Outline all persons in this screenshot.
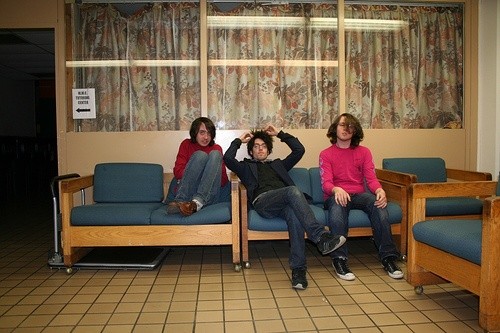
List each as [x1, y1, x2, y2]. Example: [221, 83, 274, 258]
[167, 117, 229, 217]
[222, 124, 346, 290]
[319, 113, 403, 281]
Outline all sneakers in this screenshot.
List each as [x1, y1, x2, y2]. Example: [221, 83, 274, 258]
[331, 256, 356, 281]
[316, 232, 346, 256]
[381, 257, 404, 279]
[291, 269, 308, 290]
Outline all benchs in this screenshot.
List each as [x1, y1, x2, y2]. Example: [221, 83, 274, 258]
[239, 168, 414, 270]
[58, 162, 242, 274]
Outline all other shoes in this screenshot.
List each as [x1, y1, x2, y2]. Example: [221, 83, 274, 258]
[167, 201, 181, 214]
[177, 200, 197, 216]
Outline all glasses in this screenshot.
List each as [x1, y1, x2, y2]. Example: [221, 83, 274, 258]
[253, 145, 268, 149]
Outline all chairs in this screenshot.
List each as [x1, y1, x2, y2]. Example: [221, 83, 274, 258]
[378, 158, 493, 248]
[407, 171, 500, 333]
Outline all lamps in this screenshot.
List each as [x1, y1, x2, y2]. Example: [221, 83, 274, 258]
[205, 15, 417, 32]
